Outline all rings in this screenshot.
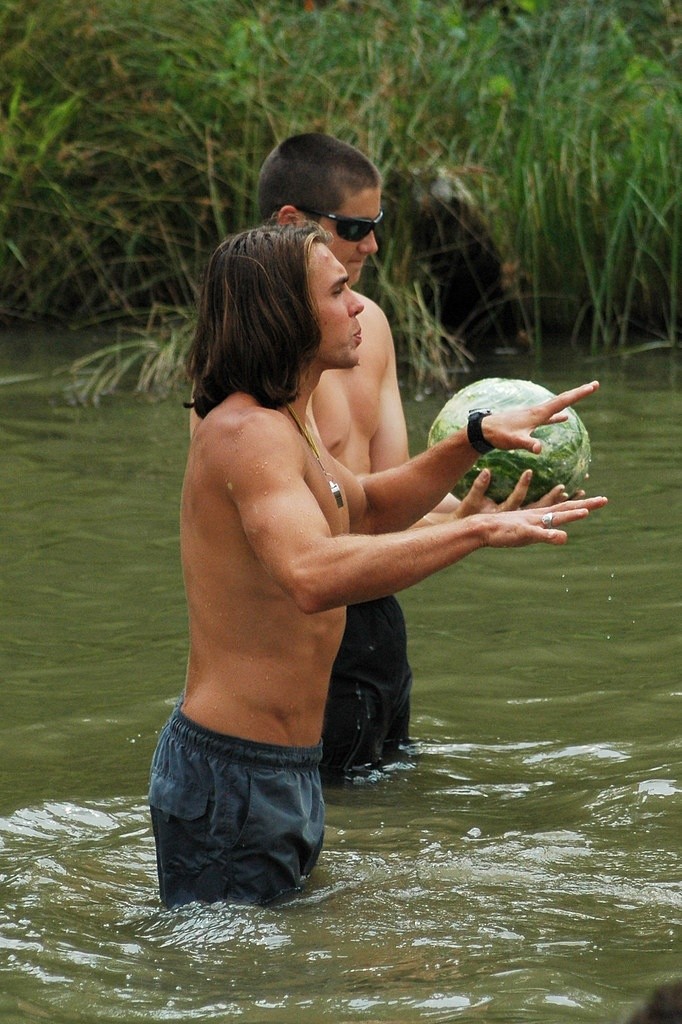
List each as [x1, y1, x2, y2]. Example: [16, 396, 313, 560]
[542, 512, 553, 529]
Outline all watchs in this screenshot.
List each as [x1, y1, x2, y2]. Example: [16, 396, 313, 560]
[466, 407, 496, 456]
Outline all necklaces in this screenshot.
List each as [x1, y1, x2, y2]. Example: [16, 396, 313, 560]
[286, 402, 344, 508]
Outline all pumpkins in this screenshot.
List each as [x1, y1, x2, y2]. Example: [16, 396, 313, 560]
[425, 379, 592, 508]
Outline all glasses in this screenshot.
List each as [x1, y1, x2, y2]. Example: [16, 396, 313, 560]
[276, 203, 383, 243]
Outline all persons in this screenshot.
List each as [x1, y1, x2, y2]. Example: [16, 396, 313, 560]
[190, 133, 587, 777]
[148, 223, 608, 910]
